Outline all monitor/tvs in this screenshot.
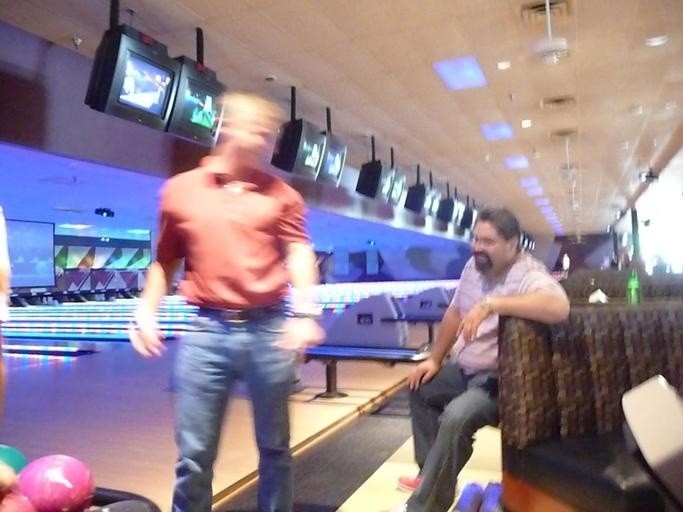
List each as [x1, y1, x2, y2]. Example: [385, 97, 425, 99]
[83, 21, 182, 131]
[270, 118, 328, 182]
[436, 199, 465, 226]
[460, 206, 478, 231]
[165, 54, 230, 149]
[519, 231, 536, 251]
[319, 130, 348, 188]
[355, 160, 406, 206]
[404, 184, 442, 217]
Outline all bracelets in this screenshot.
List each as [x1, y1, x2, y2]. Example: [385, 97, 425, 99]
[480, 296, 494, 312]
[288, 314, 318, 320]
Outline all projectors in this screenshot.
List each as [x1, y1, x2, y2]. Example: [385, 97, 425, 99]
[94, 209, 115, 218]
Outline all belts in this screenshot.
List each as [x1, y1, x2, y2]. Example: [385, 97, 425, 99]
[219, 308, 251, 324]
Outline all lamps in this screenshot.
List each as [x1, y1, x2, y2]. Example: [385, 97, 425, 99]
[521, 0, 584, 245]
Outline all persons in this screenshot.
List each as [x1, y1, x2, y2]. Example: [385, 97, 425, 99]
[397, 208, 570, 512]
[0, 206, 12, 423]
[127, 89, 318, 512]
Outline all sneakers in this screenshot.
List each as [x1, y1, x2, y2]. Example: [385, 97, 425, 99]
[398, 475, 423, 490]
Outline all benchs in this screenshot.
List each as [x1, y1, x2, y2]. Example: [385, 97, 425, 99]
[305, 345, 431, 398]
[380, 316, 442, 345]
